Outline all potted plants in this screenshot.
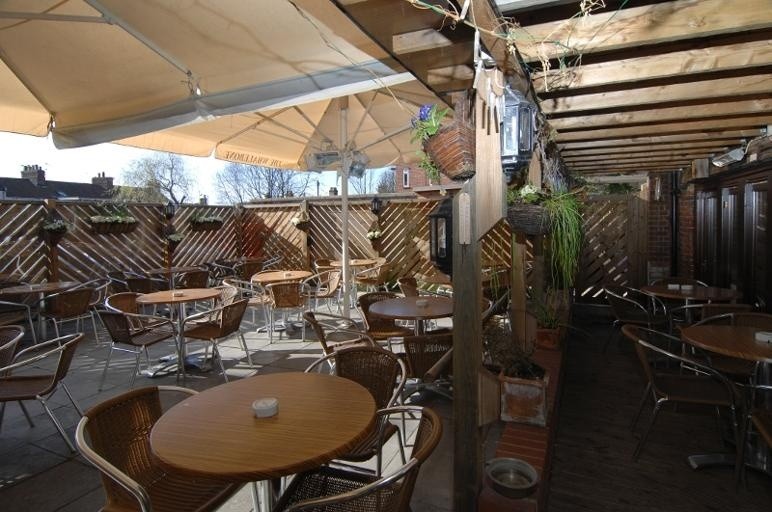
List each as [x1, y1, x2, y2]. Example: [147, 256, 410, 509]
[527, 283, 593, 350]
[484, 321, 552, 427]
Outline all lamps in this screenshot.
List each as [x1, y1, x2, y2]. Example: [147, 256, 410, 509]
[371, 194, 381, 214]
[502, 85, 534, 179]
[711, 147, 744, 169]
[428, 195, 454, 278]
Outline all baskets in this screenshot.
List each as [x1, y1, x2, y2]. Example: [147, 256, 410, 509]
[424, 97, 476, 181]
[507, 193, 562, 236]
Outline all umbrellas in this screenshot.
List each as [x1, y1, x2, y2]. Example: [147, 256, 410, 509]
[0, 1, 415, 151]
[108, 79, 450, 328]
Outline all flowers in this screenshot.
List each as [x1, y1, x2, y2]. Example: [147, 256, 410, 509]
[408, 102, 449, 195]
[43, 216, 223, 242]
[291, 217, 305, 227]
[509, 182, 587, 300]
[366, 230, 382, 240]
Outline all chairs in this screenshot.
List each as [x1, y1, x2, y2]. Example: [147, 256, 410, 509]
[176, 286, 242, 366]
[272, 405, 442, 512]
[0, 331, 81, 495]
[173, 298, 251, 386]
[95, 309, 177, 394]
[107, 289, 176, 374]
[1, 256, 354, 348]
[72, 382, 261, 512]
[354, 257, 508, 378]
[392, 332, 450, 423]
[280, 345, 407, 508]
[303, 311, 416, 420]
[604, 276, 772, 494]
